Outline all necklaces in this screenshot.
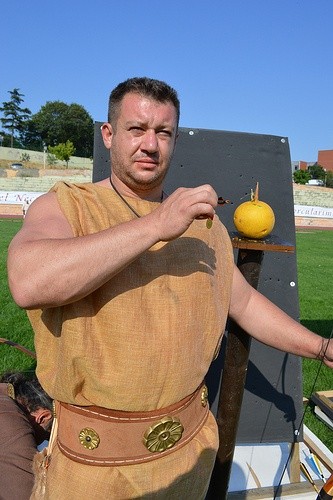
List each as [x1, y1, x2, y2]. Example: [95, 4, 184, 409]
[108, 177, 164, 219]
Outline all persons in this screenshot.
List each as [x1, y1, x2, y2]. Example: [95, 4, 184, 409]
[7, 77, 333, 500]
[0, 360, 53, 500]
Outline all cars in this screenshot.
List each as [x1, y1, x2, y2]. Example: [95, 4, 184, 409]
[10, 163, 24, 171]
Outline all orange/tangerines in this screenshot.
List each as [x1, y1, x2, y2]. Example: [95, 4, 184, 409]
[233, 180, 275, 238]
